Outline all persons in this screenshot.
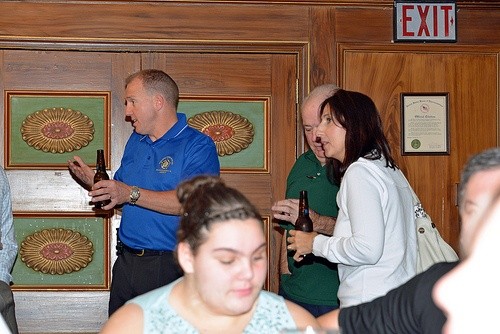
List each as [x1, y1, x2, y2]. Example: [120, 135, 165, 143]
[99, 175, 326, 334]
[271, 83, 341, 318]
[315, 147, 500, 334]
[1, 166, 20, 333]
[431, 193, 500, 333]
[287, 89, 459, 309]
[67, 69, 220, 319]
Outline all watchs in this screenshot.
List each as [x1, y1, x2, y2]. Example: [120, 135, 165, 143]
[130, 185, 141, 205]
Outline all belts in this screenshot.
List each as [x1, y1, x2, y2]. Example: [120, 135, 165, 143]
[123, 244, 160, 256]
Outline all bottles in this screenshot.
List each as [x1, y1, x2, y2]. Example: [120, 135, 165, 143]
[295, 190, 313, 257]
[93, 149, 112, 210]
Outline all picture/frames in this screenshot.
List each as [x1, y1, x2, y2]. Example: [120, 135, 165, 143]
[177, 93, 271, 174]
[4, 89, 112, 170]
[10, 211, 111, 291]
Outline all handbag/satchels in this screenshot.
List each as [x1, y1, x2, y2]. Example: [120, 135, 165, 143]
[396, 166, 460, 275]
